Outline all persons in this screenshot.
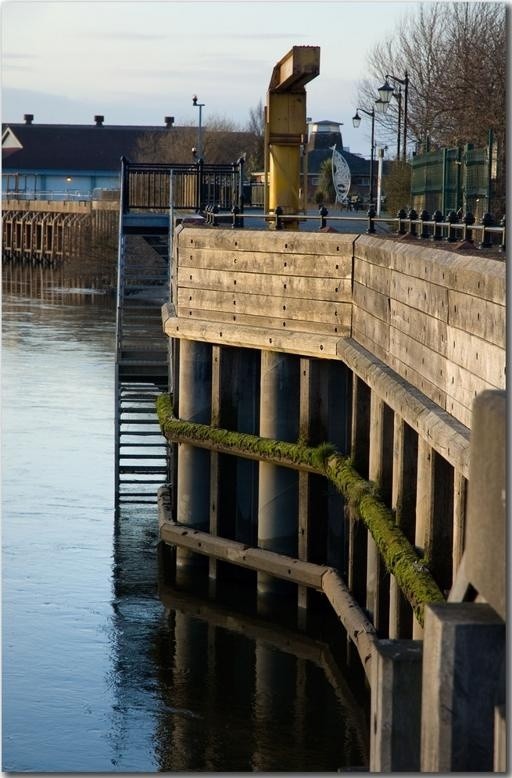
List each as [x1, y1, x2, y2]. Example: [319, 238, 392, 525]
[351, 198, 360, 213]
[346, 195, 353, 211]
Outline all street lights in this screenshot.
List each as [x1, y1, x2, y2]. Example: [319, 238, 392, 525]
[353, 107, 377, 202]
[190, 93, 205, 213]
[375, 71, 410, 170]
